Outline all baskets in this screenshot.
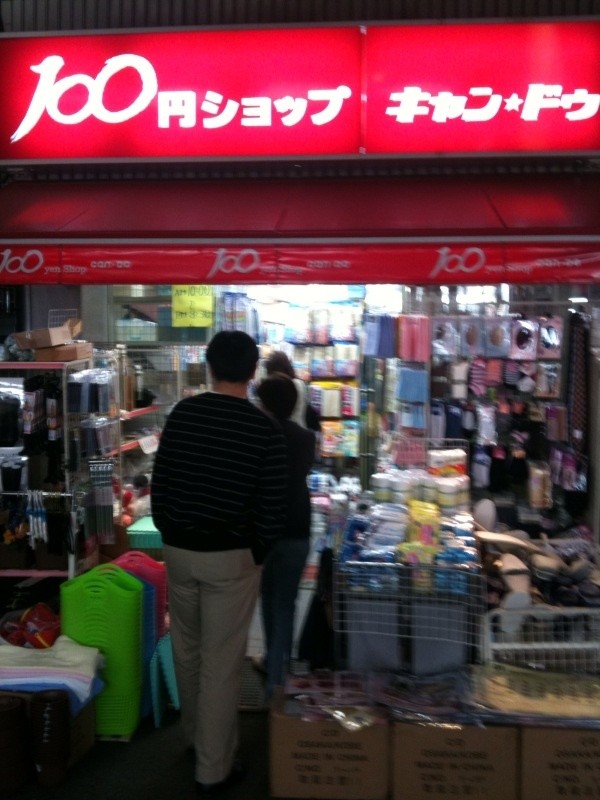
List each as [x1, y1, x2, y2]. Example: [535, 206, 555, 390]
[59, 550, 167, 736]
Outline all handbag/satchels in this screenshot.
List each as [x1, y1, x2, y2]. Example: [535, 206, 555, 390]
[303, 384, 320, 428]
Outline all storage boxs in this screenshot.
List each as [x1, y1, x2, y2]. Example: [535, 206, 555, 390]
[267, 684, 600, 800]
[15, 326, 92, 361]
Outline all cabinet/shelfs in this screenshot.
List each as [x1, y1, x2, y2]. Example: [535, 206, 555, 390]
[76, 342, 166, 559]
[0, 357, 98, 582]
[362, 314, 582, 542]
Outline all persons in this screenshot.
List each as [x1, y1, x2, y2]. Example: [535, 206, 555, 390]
[251, 351, 316, 683]
[148, 329, 289, 800]
